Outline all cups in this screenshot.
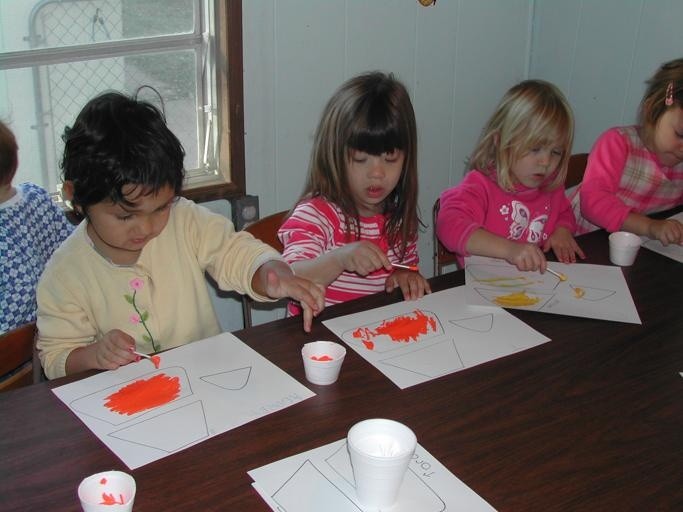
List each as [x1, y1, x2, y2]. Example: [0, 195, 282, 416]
[301, 341, 346, 386]
[78, 471, 136, 512]
[608, 232, 641, 266]
[347, 418, 417, 510]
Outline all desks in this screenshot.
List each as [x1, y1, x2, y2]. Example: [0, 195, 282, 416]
[1, 205, 682, 511]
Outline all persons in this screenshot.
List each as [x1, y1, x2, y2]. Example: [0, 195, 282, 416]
[0, 123, 76, 338]
[33, 85, 327, 382]
[564, 57, 683, 250]
[435, 76, 587, 276]
[275, 69, 433, 319]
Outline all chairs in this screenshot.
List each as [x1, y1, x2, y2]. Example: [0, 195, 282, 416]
[431, 151, 588, 278]
[239, 210, 295, 327]
